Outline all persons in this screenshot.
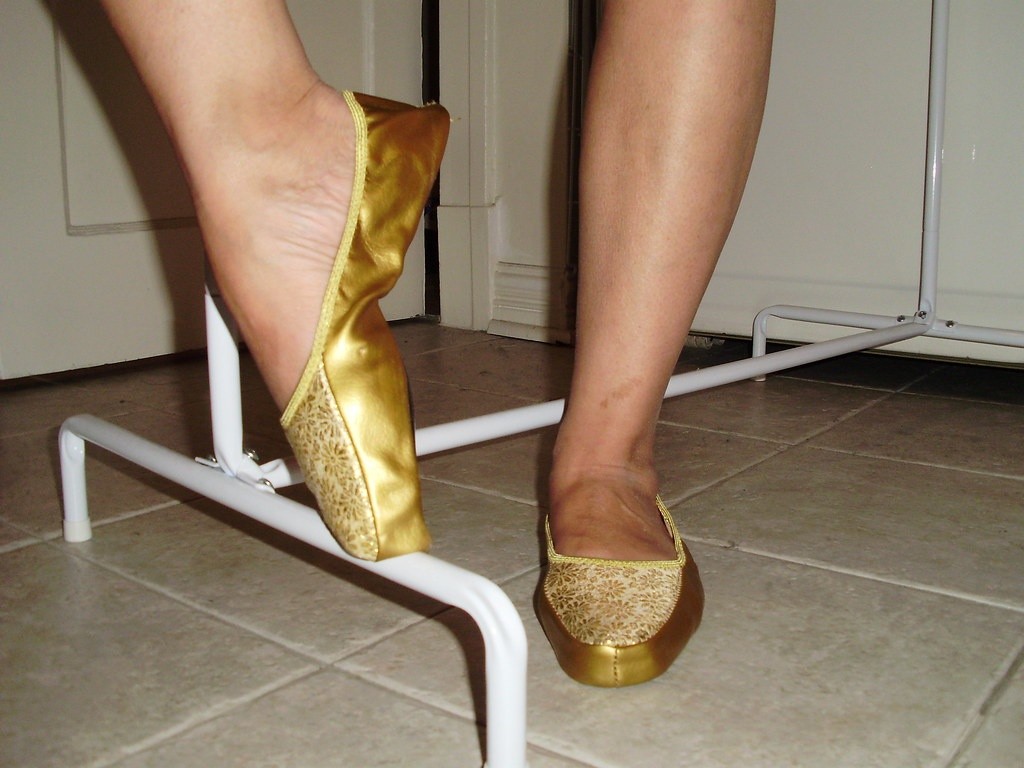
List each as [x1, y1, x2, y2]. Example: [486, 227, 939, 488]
[97, 1, 778, 688]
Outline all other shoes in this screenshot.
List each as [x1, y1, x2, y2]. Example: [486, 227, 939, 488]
[534, 485, 708, 693]
[281, 88, 452, 564]
